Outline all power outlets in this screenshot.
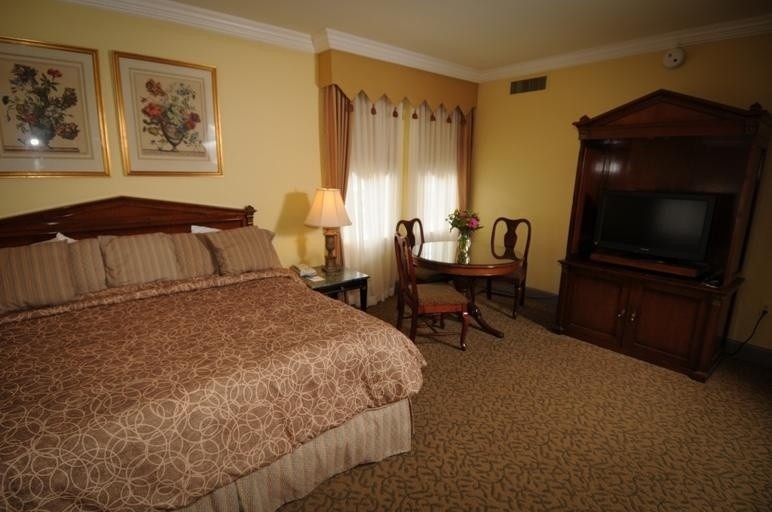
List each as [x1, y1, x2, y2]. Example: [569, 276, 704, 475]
[760, 301, 770, 317]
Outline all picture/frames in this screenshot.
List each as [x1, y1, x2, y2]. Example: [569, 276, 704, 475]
[1, 36, 111, 177]
[108, 50, 224, 176]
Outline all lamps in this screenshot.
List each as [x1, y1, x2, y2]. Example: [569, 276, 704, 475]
[304, 188, 353, 277]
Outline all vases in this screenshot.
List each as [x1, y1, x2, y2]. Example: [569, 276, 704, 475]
[458, 232, 472, 264]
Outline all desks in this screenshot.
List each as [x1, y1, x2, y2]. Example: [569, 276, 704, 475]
[410, 242, 524, 339]
[296, 268, 371, 312]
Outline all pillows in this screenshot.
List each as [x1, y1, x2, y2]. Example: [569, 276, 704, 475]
[171, 231, 219, 280]
[0, 239, 81, 316]
[207, 227, 277, 274]
[190, 224, 222, 236]
[62, 236, 114, 295]
[54, 232, 80, 245]
[98, 233, 175, 286]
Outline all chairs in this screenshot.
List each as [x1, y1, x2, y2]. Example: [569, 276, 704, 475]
[487, 217, 531, 320]
[392, 232, 470, 354]
[397, 218, 445, 330]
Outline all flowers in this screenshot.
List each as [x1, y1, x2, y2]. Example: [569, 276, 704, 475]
[450, 210, 481, 236]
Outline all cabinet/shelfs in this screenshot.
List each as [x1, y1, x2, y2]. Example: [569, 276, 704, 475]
[554, 89, 772, 384]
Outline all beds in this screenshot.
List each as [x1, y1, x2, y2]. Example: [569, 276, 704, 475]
[1, 198, 428, 511]
